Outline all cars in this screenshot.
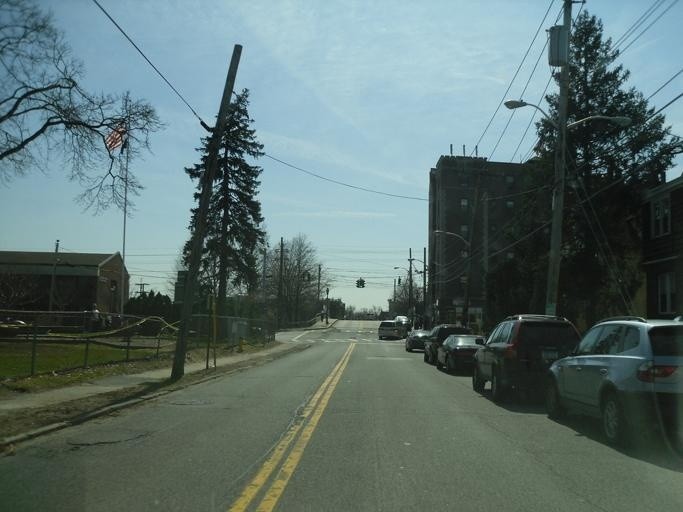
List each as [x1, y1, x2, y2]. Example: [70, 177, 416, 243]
[0, 316, 31, 338]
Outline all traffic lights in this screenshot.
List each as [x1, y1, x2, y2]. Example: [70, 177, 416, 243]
[355, 279, 360, 287]
[360, 279, 365, 288]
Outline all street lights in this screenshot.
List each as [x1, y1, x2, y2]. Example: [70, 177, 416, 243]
[503, 94, 631, 322]
[393, 254, 440, 330]
[434, 226, 497, 328]
